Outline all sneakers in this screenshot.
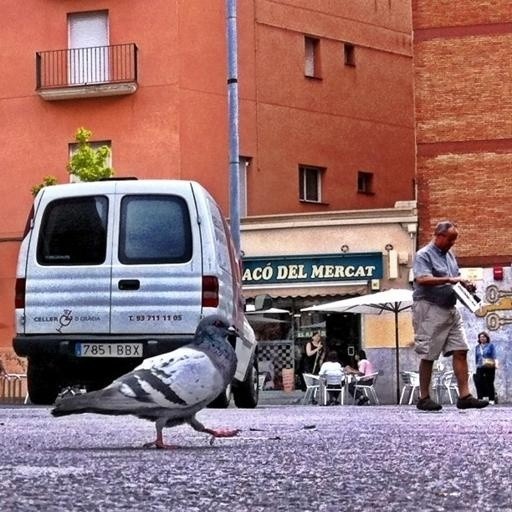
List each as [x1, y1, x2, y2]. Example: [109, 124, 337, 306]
[416, 395, 442, 411]
[357, 396, 369, 405]
[327, 399, 340, 406]
[456, 393, 490, 409]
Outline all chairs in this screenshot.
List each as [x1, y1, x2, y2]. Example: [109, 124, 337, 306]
[398, 368, 471, 405]
[301, 369, 380, 406]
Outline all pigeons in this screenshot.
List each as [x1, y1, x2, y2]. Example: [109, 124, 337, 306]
[50, 314, 244, 450]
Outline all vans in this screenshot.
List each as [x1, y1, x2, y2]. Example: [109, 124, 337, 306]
[9, 176, 262, 410]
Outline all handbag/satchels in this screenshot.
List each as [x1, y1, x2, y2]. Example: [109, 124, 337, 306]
[426, 286, 456, 307]
[482, 358, 495, 368]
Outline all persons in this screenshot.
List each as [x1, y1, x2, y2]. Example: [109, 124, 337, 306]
[411, 221, 488, 411]
[475, 330, 498, 405]
[298, 332, 373, 406]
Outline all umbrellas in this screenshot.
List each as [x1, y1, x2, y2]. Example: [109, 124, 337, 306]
[301, 288, 491, 405]
